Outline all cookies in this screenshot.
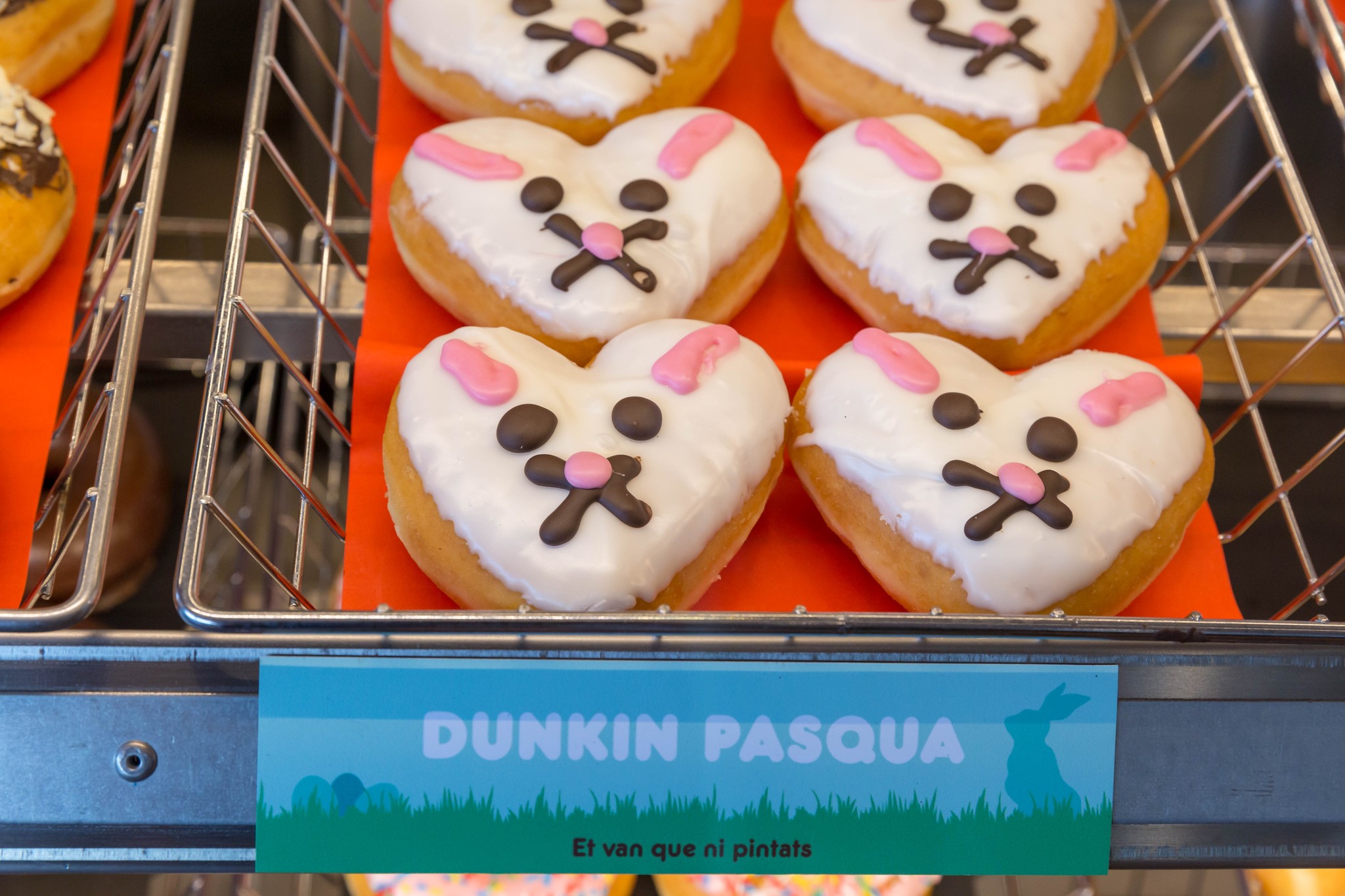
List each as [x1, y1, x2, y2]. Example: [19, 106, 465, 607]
[0, 0, 116, 312]
[381, 0, 1216, 621]
[343, 873, 947, 896]
